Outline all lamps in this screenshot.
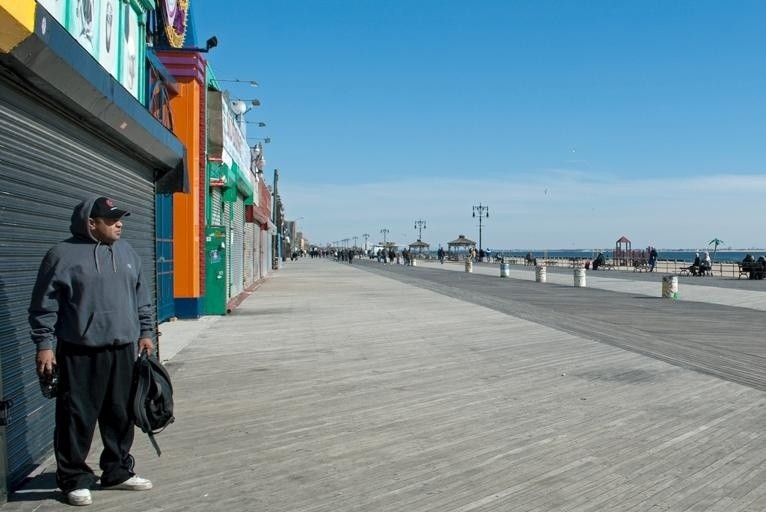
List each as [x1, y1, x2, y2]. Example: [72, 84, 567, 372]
[210, 79, 271, 144]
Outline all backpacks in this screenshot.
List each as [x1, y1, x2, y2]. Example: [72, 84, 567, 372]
[131, 347, 176, 459]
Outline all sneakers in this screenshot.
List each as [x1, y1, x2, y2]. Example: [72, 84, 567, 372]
[66, 487, 92, 506]
[100, 475, 153, 491]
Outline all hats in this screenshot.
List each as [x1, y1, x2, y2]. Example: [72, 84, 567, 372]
[89, 197, 131, 222]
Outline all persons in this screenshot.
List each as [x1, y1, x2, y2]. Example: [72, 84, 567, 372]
[698, 251, 712, 277]
[755, 256, 766, 280]
[287, 246, 538, 266]
[27, 194, 154, 505]
[689, 253, 701, 276]
[647, 246, 660, 273]
[743, 253, 756, 280]
[593, 251, 606, 270]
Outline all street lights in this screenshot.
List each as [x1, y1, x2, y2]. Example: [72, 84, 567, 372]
[472, 203, 488, 251]
[339, 227, 389, 248]
[414, 219, 426, 241]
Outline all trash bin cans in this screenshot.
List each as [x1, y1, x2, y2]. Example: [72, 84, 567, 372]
[273, 257, 282, 269]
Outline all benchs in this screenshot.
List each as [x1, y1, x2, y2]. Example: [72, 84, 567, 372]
[737, 262, 764, 280]
[680, 267, 714, 277]
